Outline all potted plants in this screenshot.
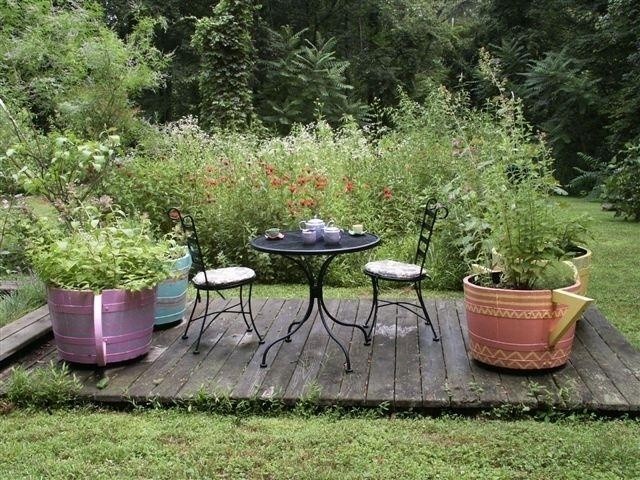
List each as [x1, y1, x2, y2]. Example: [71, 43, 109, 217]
[1, 182, 191, 370]
[434, 45, 595, 370]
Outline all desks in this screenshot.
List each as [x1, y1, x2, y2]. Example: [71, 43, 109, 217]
[250, 229, 380, 372]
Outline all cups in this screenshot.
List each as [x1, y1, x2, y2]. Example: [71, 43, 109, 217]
[301, 229, 317, 245]
[352, 225, 364, 233]
[265, 228, 281, 237]
[321, 226, 345, 245]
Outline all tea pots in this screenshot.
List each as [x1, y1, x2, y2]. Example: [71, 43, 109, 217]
[298, 214, 335, 242]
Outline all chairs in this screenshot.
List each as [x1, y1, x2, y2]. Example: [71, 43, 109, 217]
[167, 208, 265, 355]
[362, 198, 448, 341]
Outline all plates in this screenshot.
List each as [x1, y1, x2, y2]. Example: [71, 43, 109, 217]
[348, 230, 368, 236]
[264, 235, 287, 240]
